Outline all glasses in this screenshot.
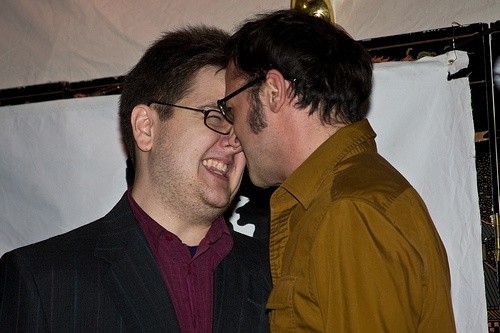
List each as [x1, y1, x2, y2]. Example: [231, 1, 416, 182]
[217, 77, 271, 121]
[145, 100, 232, 135]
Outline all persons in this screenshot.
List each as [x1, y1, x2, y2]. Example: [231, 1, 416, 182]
[224, 9, 457, 333]
[0, 24, 272, 333]
